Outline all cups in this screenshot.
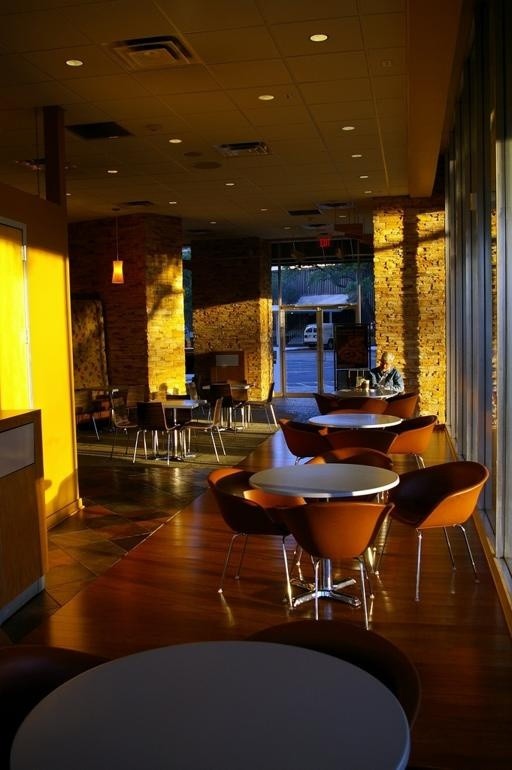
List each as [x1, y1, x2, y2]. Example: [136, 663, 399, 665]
[355, 377, 362, 388]
[362, 379, 370, 390]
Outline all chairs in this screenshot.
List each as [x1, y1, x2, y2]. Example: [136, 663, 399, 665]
[0, 643, 114, 770]
[245, 618, 424, 735]
[75, 379, 279, 469]
[312, 386, 421, 420]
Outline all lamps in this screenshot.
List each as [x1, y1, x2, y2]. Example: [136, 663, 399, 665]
[111, 215, 125, 285]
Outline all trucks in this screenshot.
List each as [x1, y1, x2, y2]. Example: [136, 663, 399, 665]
[303, 323, 333, 350]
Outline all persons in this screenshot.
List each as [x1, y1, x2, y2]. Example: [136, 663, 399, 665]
[361, 351, 404, 394]
